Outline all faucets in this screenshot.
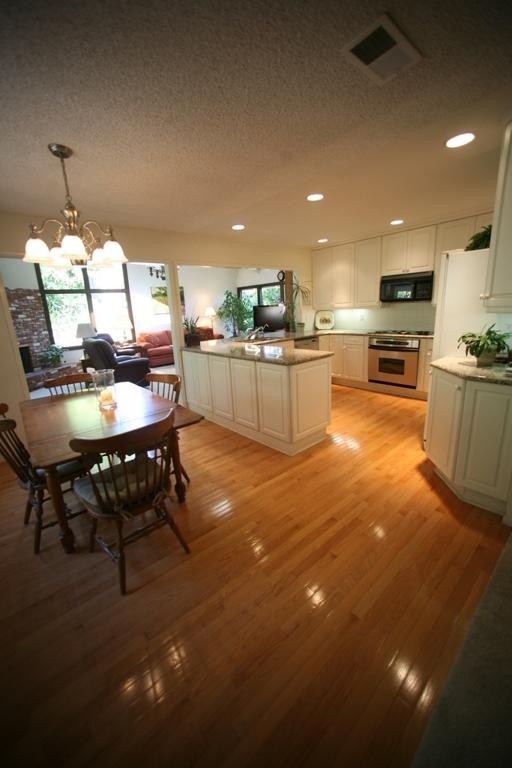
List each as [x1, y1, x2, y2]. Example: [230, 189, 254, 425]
[263, 324, 269, 329]
[247, 326, 263, 339]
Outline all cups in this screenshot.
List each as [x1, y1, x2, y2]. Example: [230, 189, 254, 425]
[91, 368, 117, 413]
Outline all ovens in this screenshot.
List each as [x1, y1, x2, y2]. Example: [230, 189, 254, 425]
[368, 336, 420, 391]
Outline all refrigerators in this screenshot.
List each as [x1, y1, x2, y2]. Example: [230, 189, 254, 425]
[433, 248, 511, 361]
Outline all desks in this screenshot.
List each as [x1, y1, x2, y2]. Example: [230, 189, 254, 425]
[18, 379, 206, 555]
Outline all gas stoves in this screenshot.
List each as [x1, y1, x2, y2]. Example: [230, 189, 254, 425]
[374, 330, 432, 335]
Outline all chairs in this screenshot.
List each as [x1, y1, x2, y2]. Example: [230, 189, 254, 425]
[82, 333, 151, 387]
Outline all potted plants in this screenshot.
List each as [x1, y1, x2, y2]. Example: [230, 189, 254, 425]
[456, 321, 511, 368]
[183, 315, 201, 346]
[279, 275, 315, 332]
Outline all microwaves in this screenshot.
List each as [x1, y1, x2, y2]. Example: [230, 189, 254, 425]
[379, 271, 434, 303]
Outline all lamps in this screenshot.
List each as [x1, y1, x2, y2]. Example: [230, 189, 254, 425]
[76, 323, 97, 355]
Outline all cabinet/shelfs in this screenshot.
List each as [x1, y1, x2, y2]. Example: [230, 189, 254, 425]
[430, 208, 494, 306]
[415, 338, 433, 401]
[355, 233, 381, 308]
[381, 221, 436, 277]
[309, 238, 355, 311]
[318, 333, 368, 391]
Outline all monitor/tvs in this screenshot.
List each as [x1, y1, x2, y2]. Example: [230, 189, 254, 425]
[253, 305, 286, 332]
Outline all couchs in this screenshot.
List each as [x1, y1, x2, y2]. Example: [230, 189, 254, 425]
[139, 325, 224, 369]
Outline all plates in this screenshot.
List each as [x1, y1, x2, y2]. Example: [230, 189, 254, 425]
[313, 310, 335, 330]
